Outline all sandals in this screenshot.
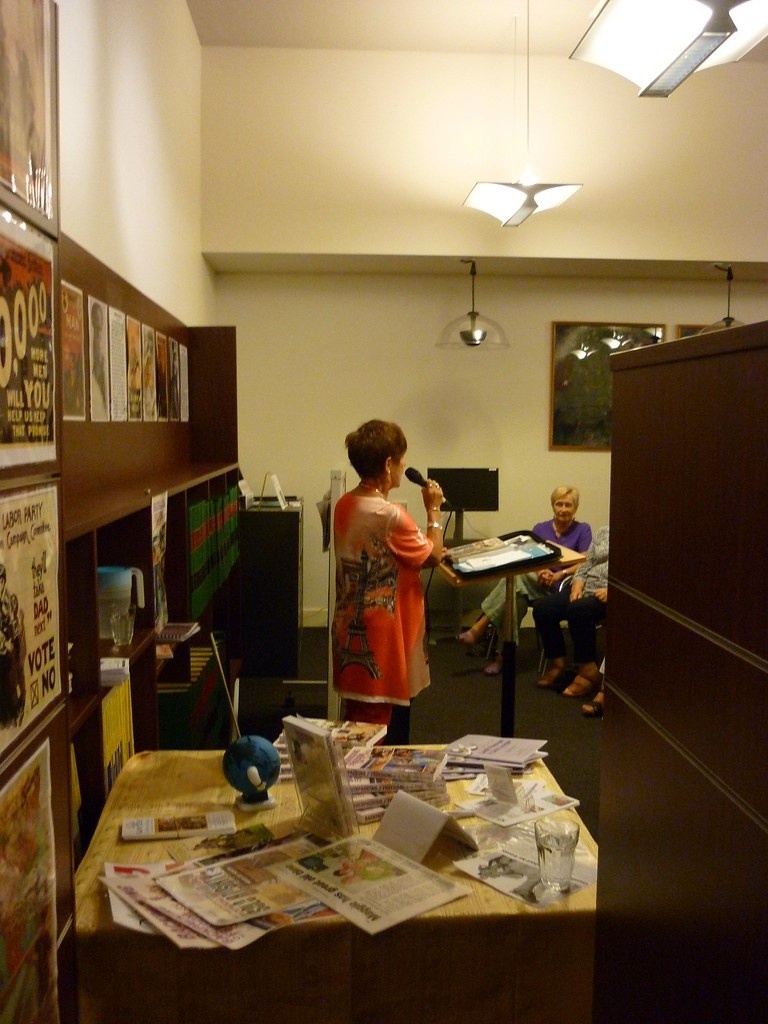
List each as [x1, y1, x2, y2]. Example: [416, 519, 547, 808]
[537, 664, 562, 689]
[582, 689, 605, 716]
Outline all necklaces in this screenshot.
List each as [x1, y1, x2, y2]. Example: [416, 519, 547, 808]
[359, 482, 384, 498]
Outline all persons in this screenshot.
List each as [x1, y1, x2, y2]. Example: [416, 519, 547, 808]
[532, 525, 610, 716]
[456, 486, 592, 677]
[331, 420, 443, 745]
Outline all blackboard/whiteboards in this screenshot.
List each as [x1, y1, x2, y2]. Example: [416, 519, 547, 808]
[328, 468, 342, 722]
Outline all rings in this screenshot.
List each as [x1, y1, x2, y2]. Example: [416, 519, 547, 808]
[426, 484, 432, 487]
[435, 483, 439, 488]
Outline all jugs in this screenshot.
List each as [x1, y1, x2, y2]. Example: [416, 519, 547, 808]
[96, 566, 145, 639]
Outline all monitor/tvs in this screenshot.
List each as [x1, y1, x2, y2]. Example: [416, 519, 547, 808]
[427, 468, 499, 511]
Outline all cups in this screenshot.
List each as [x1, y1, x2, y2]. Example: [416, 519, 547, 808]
[534, 817, 580, 892]
[108, 603, 136, 646]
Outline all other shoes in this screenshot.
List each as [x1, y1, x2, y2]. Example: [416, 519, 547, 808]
[458, 628, 480, 646]
[484, 660, 501, 674]
[562, 675, 600, 697]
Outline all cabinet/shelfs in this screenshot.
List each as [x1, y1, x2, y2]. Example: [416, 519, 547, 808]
[237, 495, 303, 677]
[58, 232, 234, 864]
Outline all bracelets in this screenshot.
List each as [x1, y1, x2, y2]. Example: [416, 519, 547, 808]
[562, 570, 567, 578]
[428, 507, 441, 514]
[427, 523, 443, 530]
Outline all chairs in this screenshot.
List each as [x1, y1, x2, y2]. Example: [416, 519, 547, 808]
[484, 574, 604, 677]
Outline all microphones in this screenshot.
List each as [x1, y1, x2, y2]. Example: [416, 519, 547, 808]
[404, 467, 452, 510]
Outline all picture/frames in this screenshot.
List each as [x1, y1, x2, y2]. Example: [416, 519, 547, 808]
[549, 322, 663, 451]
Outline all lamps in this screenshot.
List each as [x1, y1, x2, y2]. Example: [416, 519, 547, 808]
[463, 0, 583, 228]
[460, 259, 486, 346]
[568, 0, 768, 99]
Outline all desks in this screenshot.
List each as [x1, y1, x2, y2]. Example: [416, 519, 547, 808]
[71, 750, 597, 1024]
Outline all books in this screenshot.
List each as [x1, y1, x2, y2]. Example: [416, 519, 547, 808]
[273, 712, 550, 840]
